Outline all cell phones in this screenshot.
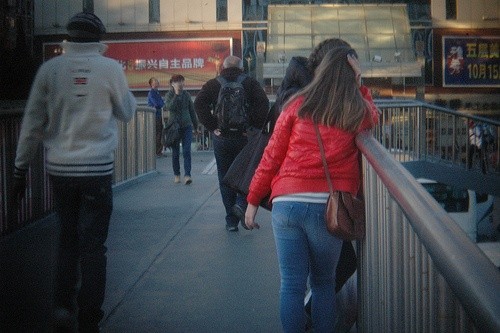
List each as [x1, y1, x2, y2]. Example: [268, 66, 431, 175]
[171, 82, 176, 87]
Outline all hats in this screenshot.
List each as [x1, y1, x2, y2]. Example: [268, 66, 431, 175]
[68, 12, 106, 38]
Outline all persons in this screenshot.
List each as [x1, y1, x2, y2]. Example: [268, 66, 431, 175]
[272, 39, 358, 333]
[462, 114, 489, 175]
[148, 77, 164, 154]
[11, 13, 137, 333]
[245, 46, 378, 333]
[165, 74, 197, 184]
[194, 56, 270, 231]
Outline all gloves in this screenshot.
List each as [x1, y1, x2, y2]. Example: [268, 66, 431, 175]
[9, 179, 26, 210]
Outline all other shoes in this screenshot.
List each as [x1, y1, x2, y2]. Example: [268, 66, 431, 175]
[174, 175, 180, 183]
[232, 202, 249, 230]
[226, 223, 238, 231]
[53, 306, 75, 329]
[184, 176, 192, 184]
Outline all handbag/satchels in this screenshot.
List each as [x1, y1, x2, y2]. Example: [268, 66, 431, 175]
[160, 122, 183, 147]
[324, 191, 365, 241]
[222, 103, 276, 212]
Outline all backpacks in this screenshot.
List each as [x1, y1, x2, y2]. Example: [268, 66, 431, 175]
[213, 75, 249, 137]
